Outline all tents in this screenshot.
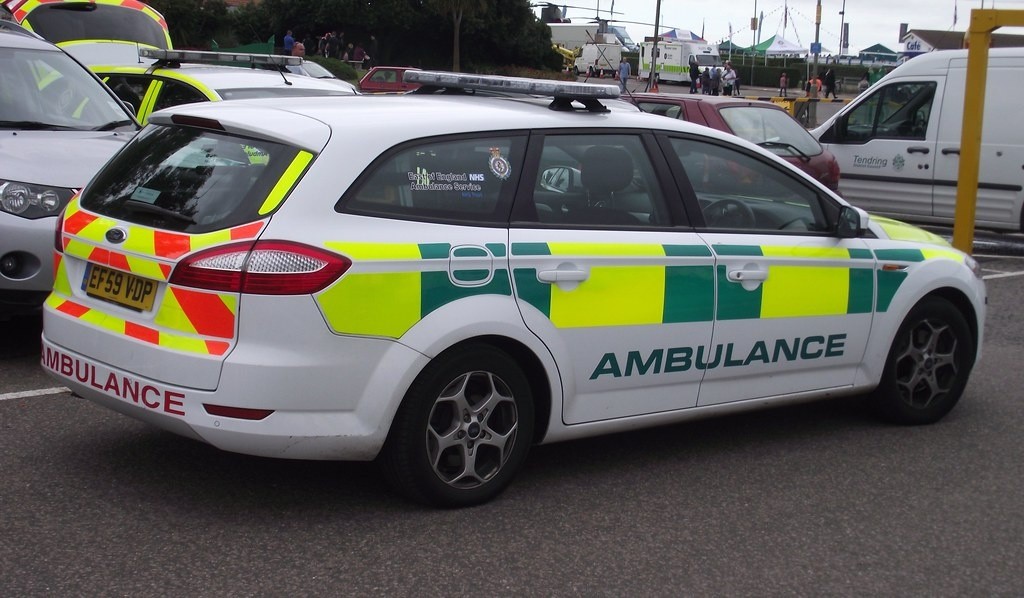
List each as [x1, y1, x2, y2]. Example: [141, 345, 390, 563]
[661, 28, 708, 43]
[718, 34, 809, 66]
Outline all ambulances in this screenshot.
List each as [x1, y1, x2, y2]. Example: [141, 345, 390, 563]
[34, 71, 990, 508]
[0, 0, 363, 174]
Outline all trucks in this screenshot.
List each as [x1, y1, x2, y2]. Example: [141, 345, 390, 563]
[638, 41, 727, 85]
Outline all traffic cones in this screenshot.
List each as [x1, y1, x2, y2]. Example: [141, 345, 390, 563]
[565, 64, 570, 73]
[599, 67, 605, 79]
[613, 68, 619, 81]
[585, 66, 590, 77]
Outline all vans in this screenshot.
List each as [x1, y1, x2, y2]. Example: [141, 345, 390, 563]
[573, 42, 622, 76]
[800, 44, 1024, 240]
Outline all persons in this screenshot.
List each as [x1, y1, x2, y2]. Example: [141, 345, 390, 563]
[805, 72, 821, 96]
[825, 68, 838, 98]
[689, 61, 740, 96]
[780, 73, 787, 97]
[858, 66, 887, 94]
[286, 42, 305, 75]
[618, 57, 631, 93]
[303, 31, 378, 69]
[284, 31, 294, 55]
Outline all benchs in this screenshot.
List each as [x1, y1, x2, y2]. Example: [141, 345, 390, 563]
[340, 60, 363, 71]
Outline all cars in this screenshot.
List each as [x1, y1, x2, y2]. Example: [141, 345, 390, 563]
[1, 19, 147, 317]
[359, 66, 427, 95]
[618, 91, 846, 208]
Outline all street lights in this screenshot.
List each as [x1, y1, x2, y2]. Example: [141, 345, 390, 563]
[838, 9, 846, 57]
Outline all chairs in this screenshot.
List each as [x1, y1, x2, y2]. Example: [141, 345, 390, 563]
[577, 146, 640, 227]
